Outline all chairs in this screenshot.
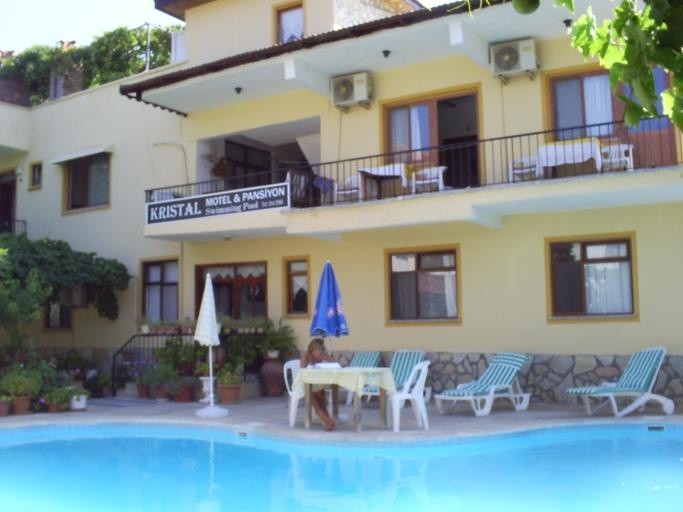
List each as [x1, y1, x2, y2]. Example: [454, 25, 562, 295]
[564, 348, 675, 419]
[434, 351, 531, 417]
[151, 169, 308, 217]
[386, 360, 431, 433]
[325, 350, 432, 409]
[284, 359, 332, 427]
[509, 137, 634, 183]
[335, 163, 448, 203]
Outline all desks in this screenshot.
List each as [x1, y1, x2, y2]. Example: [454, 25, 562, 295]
[294, 367, 397, 433]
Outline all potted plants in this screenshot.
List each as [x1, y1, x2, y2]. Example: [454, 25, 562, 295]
[0, 312, 292, 418]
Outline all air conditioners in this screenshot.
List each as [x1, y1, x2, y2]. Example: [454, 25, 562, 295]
[329, 67, 375, 111]
[487, 34, 540, 81]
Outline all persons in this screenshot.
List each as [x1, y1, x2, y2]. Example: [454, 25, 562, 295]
[301, 336, 351, 433]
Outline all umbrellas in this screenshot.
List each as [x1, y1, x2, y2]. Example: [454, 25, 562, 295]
[194, 273, 227, 417]
[310, 261, 350, 424]
